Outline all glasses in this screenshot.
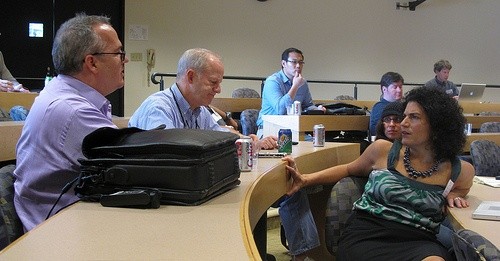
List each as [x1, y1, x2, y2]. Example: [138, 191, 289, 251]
[83, 50, 126, 64]
[384, 118, 404, 122]
[286, 60, 305, 64]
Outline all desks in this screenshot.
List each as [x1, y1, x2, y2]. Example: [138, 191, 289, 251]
[0, 116, 131, 161]
[445, 176, 500, 252]
[0, 91, 40, 117]
[462, 132, 500, 152]
[0, 140, 360, 261]
[260, 114, 500, 141]
[209, 97, 500, 119]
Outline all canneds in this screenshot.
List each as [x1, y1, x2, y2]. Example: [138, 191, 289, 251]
[234, 137, 253, 172]
[277, 128, 292, 155]
[312, 124, 325, 147]
[293, 100, 302, 115]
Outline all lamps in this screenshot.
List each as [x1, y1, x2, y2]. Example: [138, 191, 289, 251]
[396, 0, 428, 11]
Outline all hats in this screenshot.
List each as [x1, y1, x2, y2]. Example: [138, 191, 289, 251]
[382, 98, 407, 117]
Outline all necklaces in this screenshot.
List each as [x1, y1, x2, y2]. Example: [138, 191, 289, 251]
[403, 146, 440, 178]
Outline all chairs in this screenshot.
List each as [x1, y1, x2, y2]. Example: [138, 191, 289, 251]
[469, 139, 500, 177]
[325, 175, 369, 260]
[9, 105, 30, 121]
[449, 229, 500, 261]
[479, 122, 500, 132]
[0, 165, 24, 249]
[232, 88, 260, 98]
[478, 111, 500, 117]
[333, 95, 356, 100]
[241, 108, 260, 137]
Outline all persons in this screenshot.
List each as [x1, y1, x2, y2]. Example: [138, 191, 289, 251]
[0, 50, 31, 92]
[376, 101, 407, 142]
[368, 71, 404, 142]
[129, 47, 277, 261]
[205, 103, 278, 149]
[255, 47, 326, 141]
[280, 86, 475, 260]
[423, 59, 460, 100]
[13, 14, 129, 235]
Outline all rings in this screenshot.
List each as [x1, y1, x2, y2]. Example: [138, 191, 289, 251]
[292, 162, 297, 168]
[455, 196, 461, 201]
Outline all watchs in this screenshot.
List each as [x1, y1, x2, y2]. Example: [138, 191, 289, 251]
[223, 111, 232, 122]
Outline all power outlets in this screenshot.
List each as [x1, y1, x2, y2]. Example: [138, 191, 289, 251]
[131, 53, 142, 61]
